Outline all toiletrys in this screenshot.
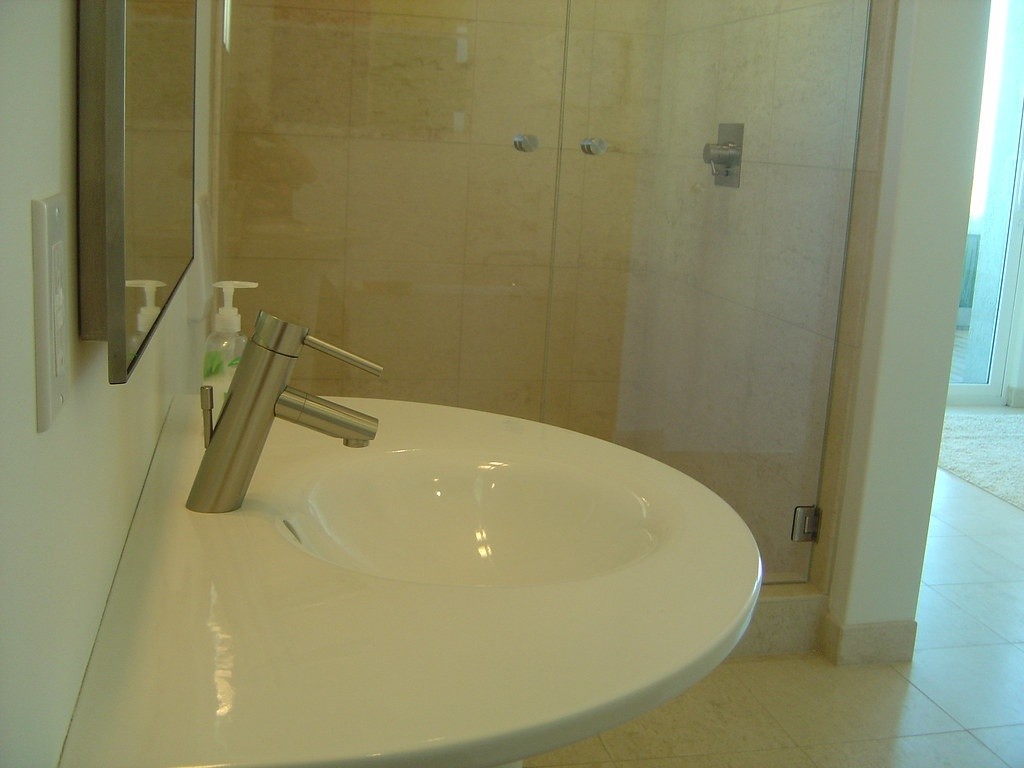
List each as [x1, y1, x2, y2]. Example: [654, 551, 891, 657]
[124, 279, 170, 368]
[199, 279, 260, 429]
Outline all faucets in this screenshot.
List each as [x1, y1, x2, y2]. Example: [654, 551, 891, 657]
[184, 308, 386, 514]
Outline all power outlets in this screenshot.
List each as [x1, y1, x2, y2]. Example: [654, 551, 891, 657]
[33, 191, 70, 433]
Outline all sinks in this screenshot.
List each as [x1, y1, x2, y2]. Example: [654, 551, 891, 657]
[282, 445, 661, 592]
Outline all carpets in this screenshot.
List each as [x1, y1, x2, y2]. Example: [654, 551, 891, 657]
[937, 412, 1023, 514]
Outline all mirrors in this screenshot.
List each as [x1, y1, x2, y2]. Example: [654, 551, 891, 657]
[79, 0, 197, 388]
